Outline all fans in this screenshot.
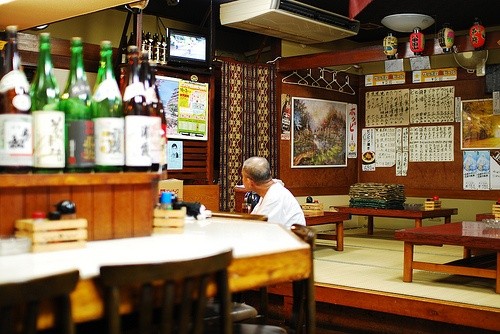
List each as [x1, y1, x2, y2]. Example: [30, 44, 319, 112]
[453, 50, 489, 76]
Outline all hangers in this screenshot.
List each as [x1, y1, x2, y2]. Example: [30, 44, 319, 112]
[282, 68, 356, 95]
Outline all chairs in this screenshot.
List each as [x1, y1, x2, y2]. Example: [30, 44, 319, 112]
[0, 251, 255, 334]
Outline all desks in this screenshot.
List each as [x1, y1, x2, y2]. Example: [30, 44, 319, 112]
[0, 168, 500, 334]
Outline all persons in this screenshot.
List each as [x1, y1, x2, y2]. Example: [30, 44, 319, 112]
[241, 157, 306, 229]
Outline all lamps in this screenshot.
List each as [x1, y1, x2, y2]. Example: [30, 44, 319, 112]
[381, 0, 435, 32]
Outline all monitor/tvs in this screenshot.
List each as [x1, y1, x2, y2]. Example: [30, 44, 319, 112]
[167, 28, 208, 67]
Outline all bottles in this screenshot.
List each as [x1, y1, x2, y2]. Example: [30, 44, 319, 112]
[138, 50, 169, 172]
[0, 26, 36, 175]
[60, 36, 97, 174]
[29, 33, 71, 175]
[128, 30, 174, 66]
[90, 39, 126, 172]
[119, 45, 157, 172]
[159, 192, 173, 211]
[31, 199, 78, 251]
[306, 196, 318, 203]
